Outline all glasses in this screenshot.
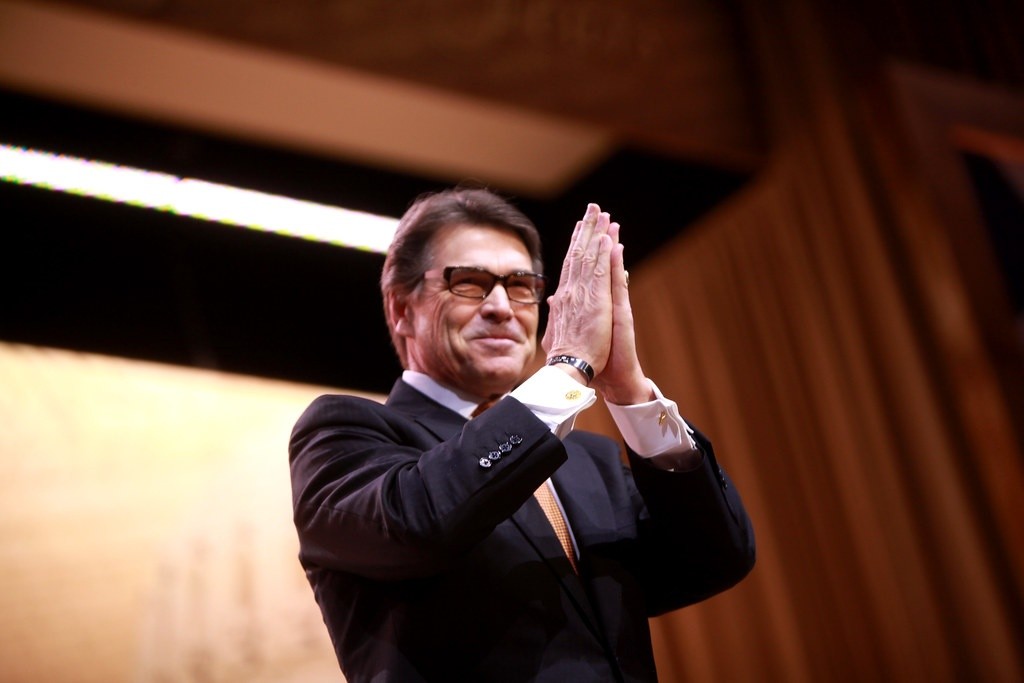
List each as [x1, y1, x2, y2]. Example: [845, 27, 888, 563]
[406, 266, 544, 303]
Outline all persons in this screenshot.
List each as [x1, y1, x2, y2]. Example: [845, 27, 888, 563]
[289, 191, 756, 683]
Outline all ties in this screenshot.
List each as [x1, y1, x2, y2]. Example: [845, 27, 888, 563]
[472, 399, 579, 576]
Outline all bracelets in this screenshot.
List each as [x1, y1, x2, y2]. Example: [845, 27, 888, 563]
[545, 355, 595, 386]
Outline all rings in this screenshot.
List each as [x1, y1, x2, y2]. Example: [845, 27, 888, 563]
[625, 270, 629, 284]
